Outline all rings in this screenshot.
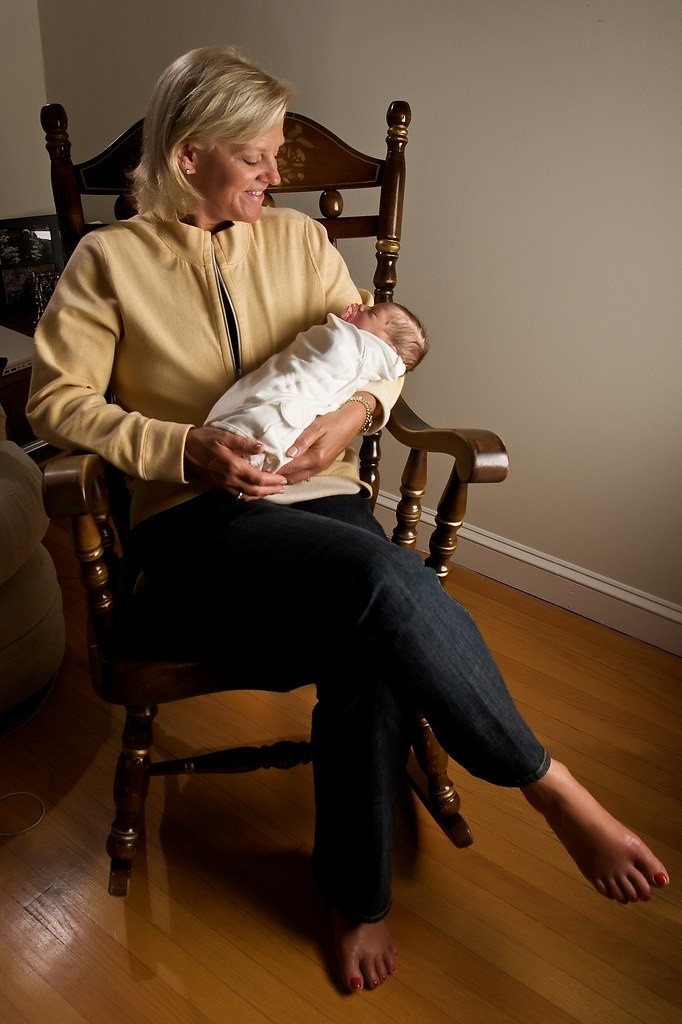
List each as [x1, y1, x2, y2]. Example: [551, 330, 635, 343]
[235, 491, 243, 500]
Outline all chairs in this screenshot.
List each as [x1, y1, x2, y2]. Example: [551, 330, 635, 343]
[41, 101, 511, 861]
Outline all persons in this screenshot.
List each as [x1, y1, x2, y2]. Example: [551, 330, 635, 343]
[200, 300, 430, 476]
[23, 43, 672, 994]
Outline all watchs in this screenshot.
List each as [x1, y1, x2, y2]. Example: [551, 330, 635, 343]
[346, 396, 373, 436]
